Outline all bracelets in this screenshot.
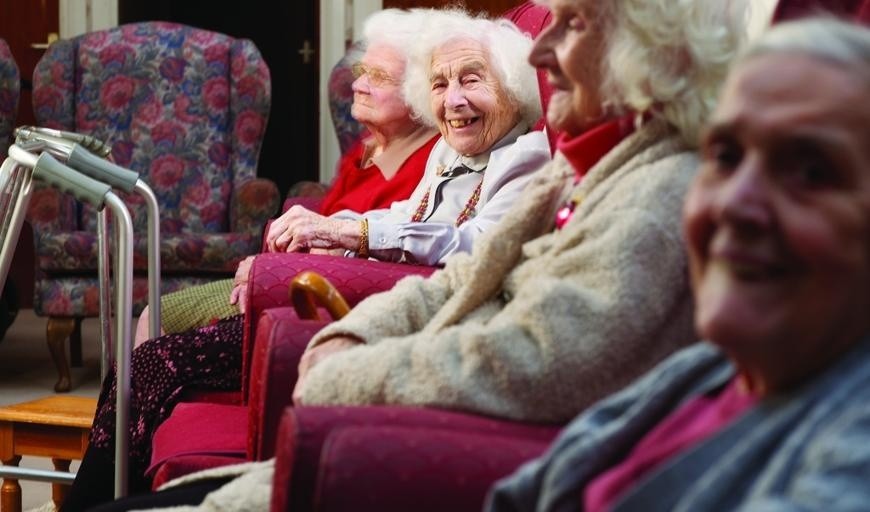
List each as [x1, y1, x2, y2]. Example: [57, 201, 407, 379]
[360, 215, 370, 258]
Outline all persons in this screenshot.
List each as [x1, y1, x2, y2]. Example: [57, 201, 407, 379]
[100, 1, 745, 511]
[61, 19, 551, 512]
[485, 15, 870, 512]
[135, 8, 442, 348]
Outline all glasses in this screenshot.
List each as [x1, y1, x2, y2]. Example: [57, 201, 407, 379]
[352, 62, 406, 86]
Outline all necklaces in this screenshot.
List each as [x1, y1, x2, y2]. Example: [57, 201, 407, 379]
[556, 198, 575, 231]
[408, 185, 485, 228]
